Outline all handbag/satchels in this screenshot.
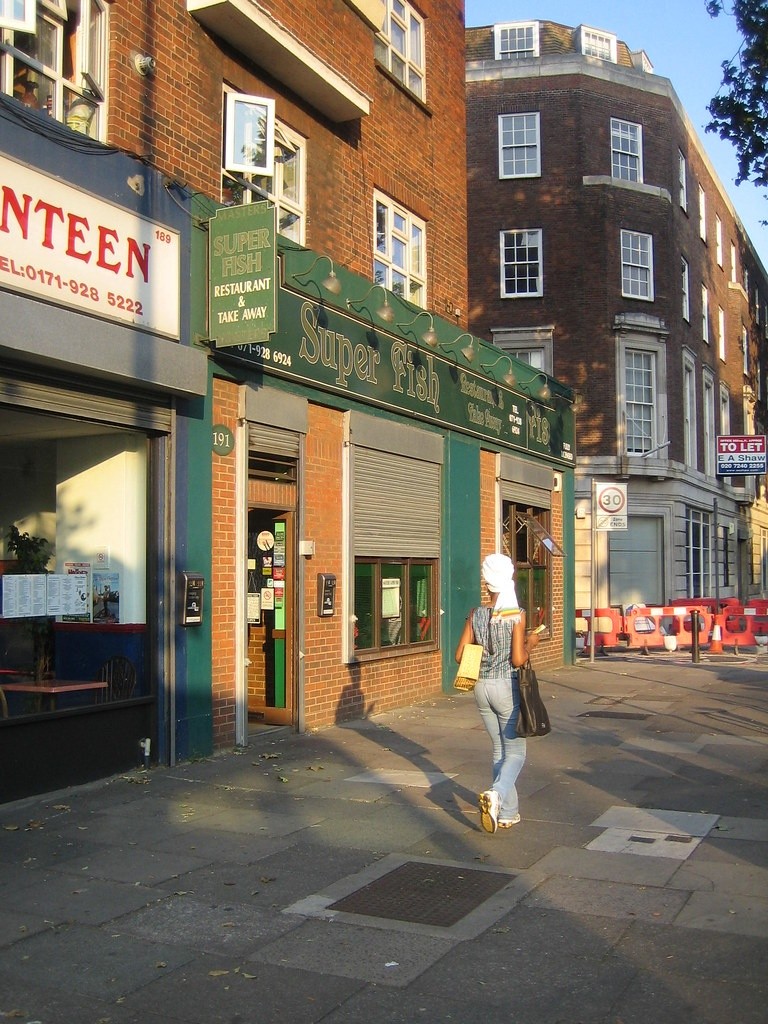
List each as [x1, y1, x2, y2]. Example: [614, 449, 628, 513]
[454, 644, 483, 691]
[514, 651, 552, 737]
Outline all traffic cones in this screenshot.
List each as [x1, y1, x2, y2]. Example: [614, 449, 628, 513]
[707, 618, 726, 655]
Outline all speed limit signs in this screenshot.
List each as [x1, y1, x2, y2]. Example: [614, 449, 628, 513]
[595, 482, 628, 532]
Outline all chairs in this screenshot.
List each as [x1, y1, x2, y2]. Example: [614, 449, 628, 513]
[92, 656, 136, 703]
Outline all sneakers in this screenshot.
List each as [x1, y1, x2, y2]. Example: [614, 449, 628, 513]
[498, 813, 521, 828]
[479, 791, 499, 834]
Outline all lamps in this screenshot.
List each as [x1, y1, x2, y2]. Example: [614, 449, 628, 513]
[518, 373, 551, 400]
[554, 393, 582, 413]
[346, 285, 395, 324]
[481, 358, 515, 386]
[439, 333, 475, 363]
[396, 312, 438, 347]
[292, 255, 341, 295]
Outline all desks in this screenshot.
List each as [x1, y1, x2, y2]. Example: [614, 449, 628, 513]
[1, 680, 108, 713]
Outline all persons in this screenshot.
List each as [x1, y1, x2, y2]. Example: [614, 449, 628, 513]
[454, 553, 540, 833]
[103, 585, 109, 617]
